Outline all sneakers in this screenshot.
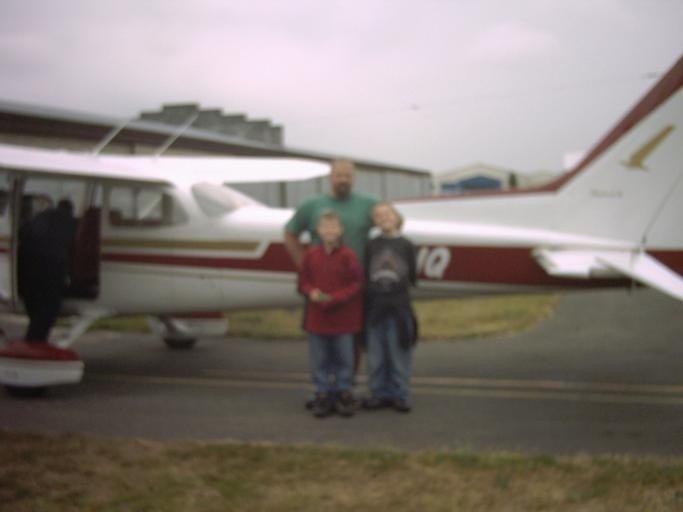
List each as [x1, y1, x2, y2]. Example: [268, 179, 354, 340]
[304, 390, 356, 417]
[392, 398, 410, 411]
[362, 395, 392, 409]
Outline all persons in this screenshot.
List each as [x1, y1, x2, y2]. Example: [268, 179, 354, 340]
[356, 197, 420, 414]
[17, 199, 77, 346]
[281, 157, 385, 412]
[292, 209, 366, 420]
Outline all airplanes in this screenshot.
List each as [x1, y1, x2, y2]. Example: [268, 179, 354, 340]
[0, 52, 683, 398]
